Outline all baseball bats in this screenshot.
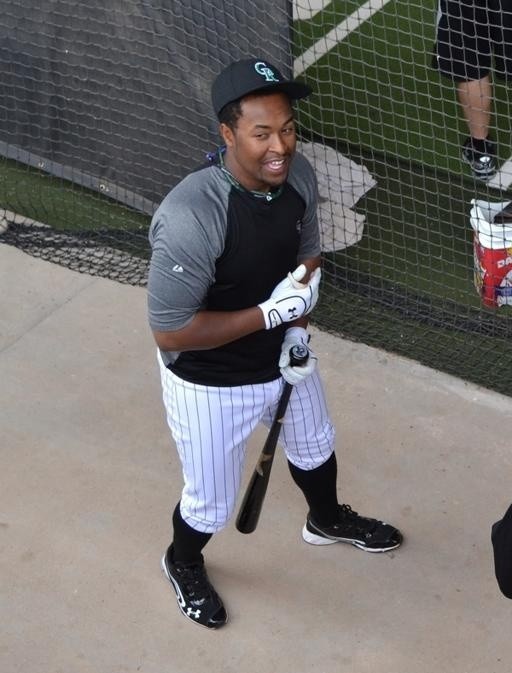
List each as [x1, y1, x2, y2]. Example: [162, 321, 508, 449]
[236, 345, 309, 534]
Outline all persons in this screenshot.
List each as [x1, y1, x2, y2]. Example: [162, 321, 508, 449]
[430, 0, 512, 175]
[146, 56, 403, 628]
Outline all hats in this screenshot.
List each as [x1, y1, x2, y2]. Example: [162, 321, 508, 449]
[211, 58, 313, 113]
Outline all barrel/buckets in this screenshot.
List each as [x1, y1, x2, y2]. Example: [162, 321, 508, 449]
[469, 198, 512, 308]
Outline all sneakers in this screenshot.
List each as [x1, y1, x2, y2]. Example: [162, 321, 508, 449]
[161, 542, 228, 628]
[461, 137, 496, 173]
[302, 504, 404, 552]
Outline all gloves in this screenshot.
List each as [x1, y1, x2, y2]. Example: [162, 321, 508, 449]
[279, 327, 318, 385]
[258, 263, 322, 330]
